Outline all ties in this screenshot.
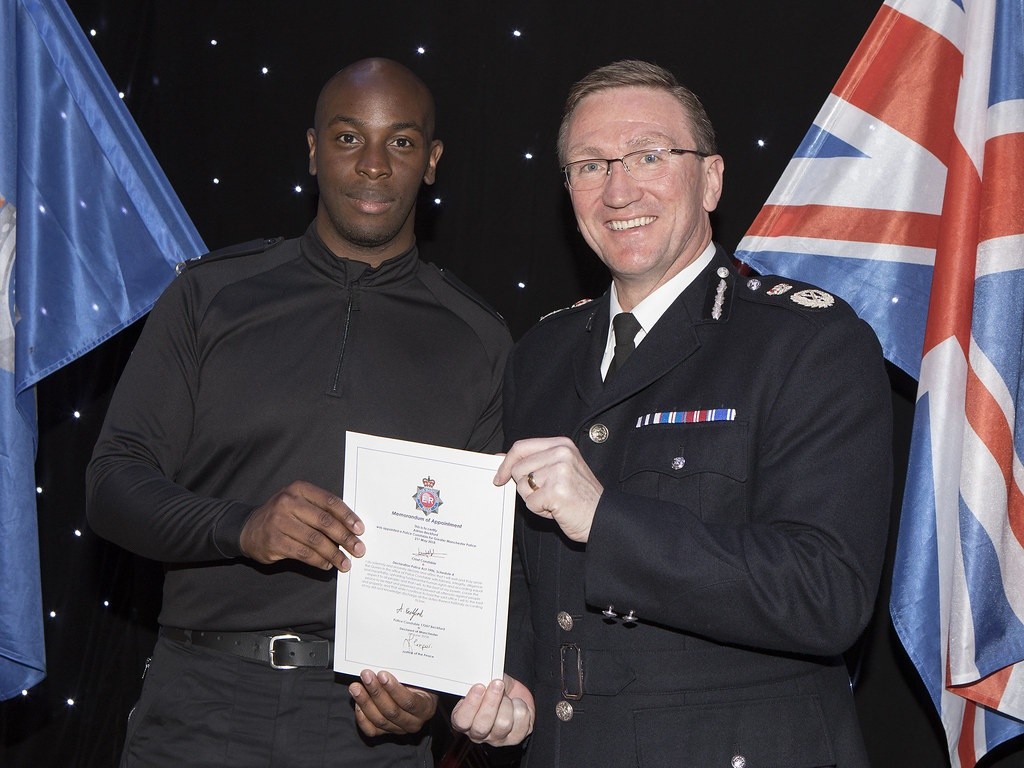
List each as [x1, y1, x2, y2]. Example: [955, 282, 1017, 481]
[603, 312, 642, 387]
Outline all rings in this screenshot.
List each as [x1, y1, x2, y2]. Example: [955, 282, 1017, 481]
[528, 472, 538, 491]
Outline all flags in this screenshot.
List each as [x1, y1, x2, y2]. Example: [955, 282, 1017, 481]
[734, 0, 1024, 768]
[0, 0, 210, 702]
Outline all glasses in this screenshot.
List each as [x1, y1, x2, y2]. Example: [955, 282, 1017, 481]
[562, 148, 712, 191]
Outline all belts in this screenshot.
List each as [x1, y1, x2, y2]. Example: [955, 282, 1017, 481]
[532, 643, 838, 701]
[161, 626, 335, 670]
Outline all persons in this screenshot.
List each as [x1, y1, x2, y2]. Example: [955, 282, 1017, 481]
[450, 61, 894, 768]
[84, 59, 516, 767]
